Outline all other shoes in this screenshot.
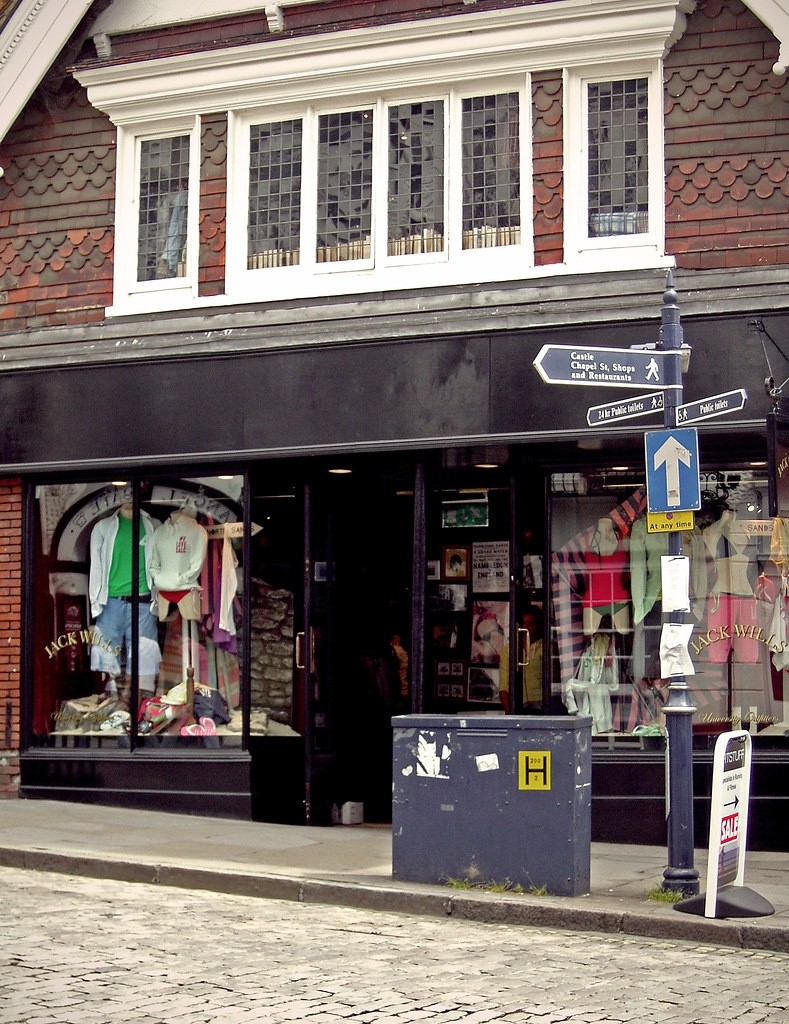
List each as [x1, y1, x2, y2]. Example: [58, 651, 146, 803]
[249, 706, 270, 736]
[226, 709, 243, 736]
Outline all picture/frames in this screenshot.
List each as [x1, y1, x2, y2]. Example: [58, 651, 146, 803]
[434, 660, 467, 701]
[441, 544, 470, 581]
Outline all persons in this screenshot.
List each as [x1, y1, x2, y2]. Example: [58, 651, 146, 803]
[89, 503, 208, 675]
[579, 511, 760, 681]
[499, 604, 543, 715]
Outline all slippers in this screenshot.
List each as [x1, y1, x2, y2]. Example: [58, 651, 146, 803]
[200, 716, 217, 735]
[181, 723, 206, 736]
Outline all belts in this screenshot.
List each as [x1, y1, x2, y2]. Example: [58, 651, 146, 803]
[114, 594, 151, 603]
